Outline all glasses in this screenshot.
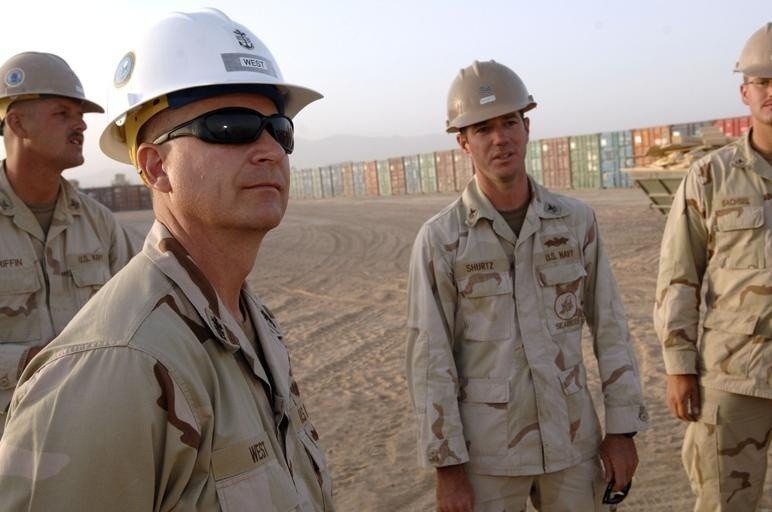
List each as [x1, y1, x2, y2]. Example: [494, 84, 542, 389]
[742, 78, 772, 86]
[602, 478, 632, 504]
[151, 107, 295, 154]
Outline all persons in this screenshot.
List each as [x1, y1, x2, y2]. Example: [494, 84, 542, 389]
[653, 23, 772, 512]
[0, 51, 140, 445]
[406, 60, 649, 512]
[0, 7, 333, 512]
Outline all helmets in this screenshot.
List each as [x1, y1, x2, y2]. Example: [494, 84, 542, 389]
[0, 50, 105, 114]
[445, 59, 537, 134]
[97, 7, 324, 163]
[732, 21, 772, 77]
